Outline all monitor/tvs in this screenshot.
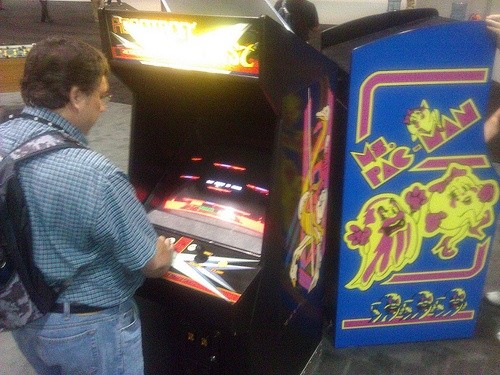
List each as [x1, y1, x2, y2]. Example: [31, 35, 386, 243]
[143, 138, 272, 257]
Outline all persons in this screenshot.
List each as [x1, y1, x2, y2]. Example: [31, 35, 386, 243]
[274, 0, 352, 101]
[90, 0, 122, 23]
[38, 0, 52, 23]
[0, 36, 173, 375]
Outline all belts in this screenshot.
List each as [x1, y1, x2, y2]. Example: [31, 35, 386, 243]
[51, 302, 116, 313]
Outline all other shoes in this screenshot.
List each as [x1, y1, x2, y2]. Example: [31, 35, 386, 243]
[486, 291, 500, 306]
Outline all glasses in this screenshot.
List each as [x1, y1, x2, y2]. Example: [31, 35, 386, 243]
[89, 85, 113, 105]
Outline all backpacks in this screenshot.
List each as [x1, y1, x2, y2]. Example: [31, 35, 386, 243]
[0, 129, 90, 330]
